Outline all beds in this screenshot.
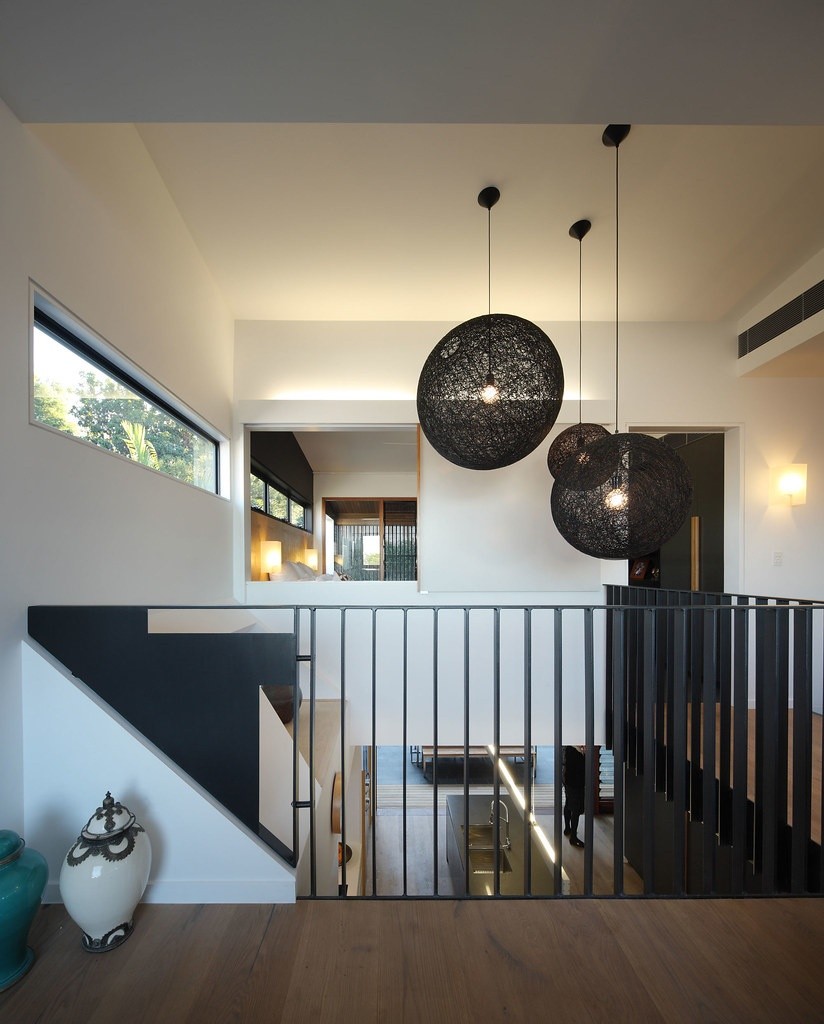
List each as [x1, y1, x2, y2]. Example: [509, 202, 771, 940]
[268, 559, 342, 582]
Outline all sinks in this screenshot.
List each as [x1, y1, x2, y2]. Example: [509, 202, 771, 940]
[468, 847, 514, 874]
[460, 823, 500, 845]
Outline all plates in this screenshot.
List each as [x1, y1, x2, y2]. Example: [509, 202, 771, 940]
[339, 842, 352, 868]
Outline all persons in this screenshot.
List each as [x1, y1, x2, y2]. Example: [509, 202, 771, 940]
[561, 746, 586, 848]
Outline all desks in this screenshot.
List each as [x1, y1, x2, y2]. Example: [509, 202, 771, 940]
[422, 748, 536, 779]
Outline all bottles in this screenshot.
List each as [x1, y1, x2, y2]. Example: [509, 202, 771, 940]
[59, 792, 152, 952]
[0, 828, 47, 995]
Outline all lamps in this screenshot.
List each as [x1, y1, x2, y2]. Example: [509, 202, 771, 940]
[305, 548, 318, 570]
[550, 125, 693, 560]
[486, 745, 573, 895]
[261, 541, 282, 573]
[416, 186, 565, 471]
[546, 218, 619, 492]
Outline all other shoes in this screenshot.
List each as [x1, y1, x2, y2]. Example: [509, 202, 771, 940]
[564, 828, 572, 835]
[569, 838, 584, 848]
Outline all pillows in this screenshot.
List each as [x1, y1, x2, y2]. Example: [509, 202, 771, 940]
[269, 560, 318, 581]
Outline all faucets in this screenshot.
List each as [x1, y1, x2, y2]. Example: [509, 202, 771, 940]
[488, 799, 512, 850]
